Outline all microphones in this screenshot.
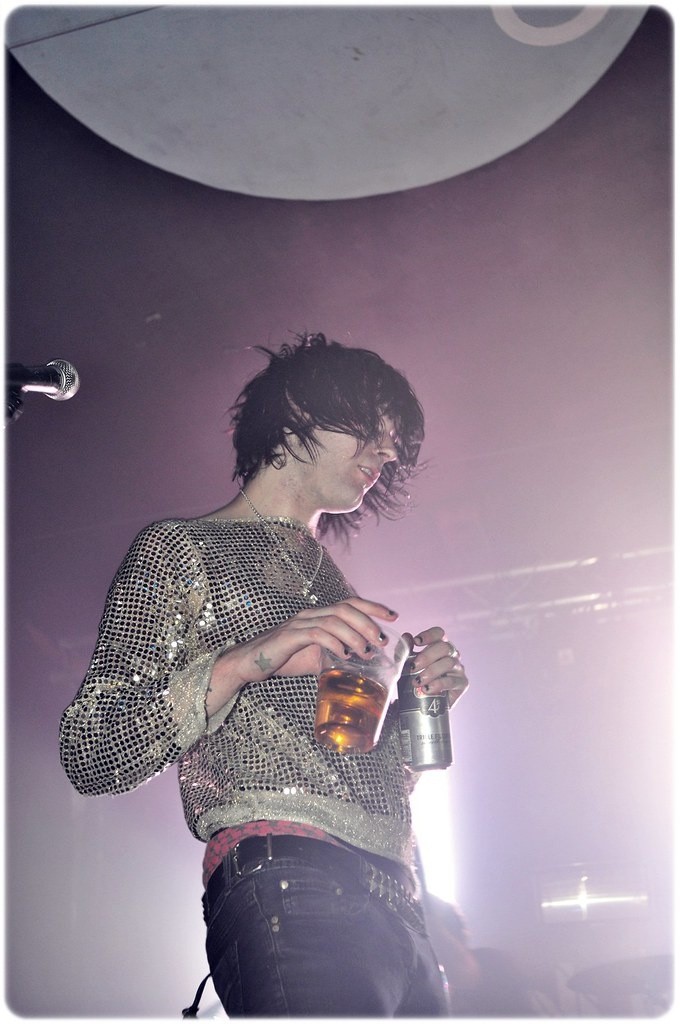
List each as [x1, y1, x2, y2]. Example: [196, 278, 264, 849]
[6, 359, 81, 402]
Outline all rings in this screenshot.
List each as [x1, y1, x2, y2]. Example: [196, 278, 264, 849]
[447, 641, 458, 657]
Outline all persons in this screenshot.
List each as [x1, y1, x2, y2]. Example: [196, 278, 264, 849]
[54, 335, 473, 1019]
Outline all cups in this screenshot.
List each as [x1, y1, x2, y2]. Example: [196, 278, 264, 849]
[314, 624, 410, 753]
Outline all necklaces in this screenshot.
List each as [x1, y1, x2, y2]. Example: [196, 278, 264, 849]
[239, 487, 324, 605]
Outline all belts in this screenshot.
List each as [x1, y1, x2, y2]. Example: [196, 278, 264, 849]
[203, 832, 428, 936]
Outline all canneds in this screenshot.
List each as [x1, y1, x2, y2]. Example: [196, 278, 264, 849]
[396, 654, 453, 772]
